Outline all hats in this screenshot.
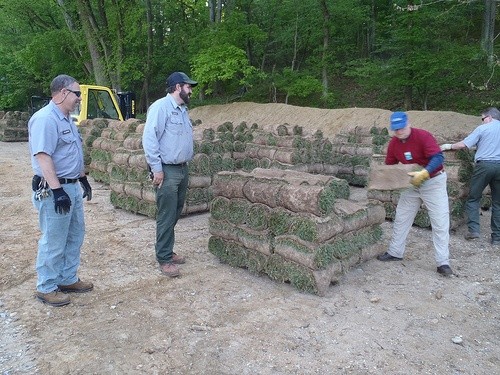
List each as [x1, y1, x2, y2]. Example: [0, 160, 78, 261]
[389, 112, 408, 131]
[166, 72, 198, 88]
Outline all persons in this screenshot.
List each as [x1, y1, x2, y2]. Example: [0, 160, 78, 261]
[377, 111, 452, 275]
[142, 72, 198, 276]
[441, 107, 500, 245]
[29, 75, 94, 307]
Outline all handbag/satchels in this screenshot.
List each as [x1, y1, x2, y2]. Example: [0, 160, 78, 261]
[32, 175, 41, 192]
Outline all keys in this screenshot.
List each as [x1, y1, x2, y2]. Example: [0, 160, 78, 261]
[34, 187, 52, 201]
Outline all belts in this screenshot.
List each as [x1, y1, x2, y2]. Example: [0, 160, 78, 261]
[58, 178, 78, 184]
[430, 169, 444, 179]
[162, 162, 187, 166]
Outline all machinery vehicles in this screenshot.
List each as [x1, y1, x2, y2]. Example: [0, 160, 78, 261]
[64, 85, 134, 132]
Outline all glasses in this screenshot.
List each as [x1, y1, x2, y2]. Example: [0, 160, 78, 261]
[66, 89, 81, 98]
[482, 116, 488, 121]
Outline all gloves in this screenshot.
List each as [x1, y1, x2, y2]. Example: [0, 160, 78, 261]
[79, 176, 92, 201]
[439, 144, 453, 151]
[50, 187, 71, 215]
[408, 169, 429, 185]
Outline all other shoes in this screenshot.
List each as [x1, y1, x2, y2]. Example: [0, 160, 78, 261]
[36, 288, 71, 307]
[57, 278, 94, 292]
[465, 232, 479, 239]
[492, 240, 500, 245]
[168, 252, 185, 264]
[437, 265, 454, 275]
[377, 252, 403, 262]
[159, 262, 179, 276]
